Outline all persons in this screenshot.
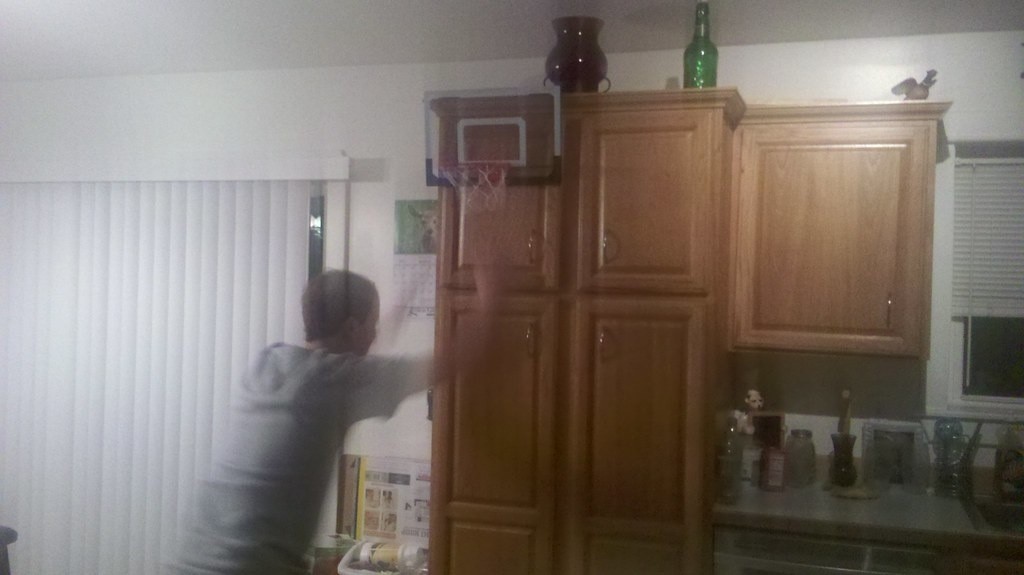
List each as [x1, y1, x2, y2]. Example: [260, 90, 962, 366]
[170, 269, 497, 575]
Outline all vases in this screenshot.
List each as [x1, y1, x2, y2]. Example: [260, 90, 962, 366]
[543, 16, 610, 94]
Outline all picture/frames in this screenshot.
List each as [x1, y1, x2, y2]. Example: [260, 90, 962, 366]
[863, 417, 925, 493]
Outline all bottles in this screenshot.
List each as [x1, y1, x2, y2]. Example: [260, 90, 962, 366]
[716, 416, 744, 507]
[829, 434, 857, 487]
[784, 429, 818, 489]
[683, 1, 720, 88]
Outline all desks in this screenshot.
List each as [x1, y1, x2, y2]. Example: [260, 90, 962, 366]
[710, 453, 1024, 575]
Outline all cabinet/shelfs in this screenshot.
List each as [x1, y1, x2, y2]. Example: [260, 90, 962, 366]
[428, 88, 746, 575]
[726, 100, 953, 360]
